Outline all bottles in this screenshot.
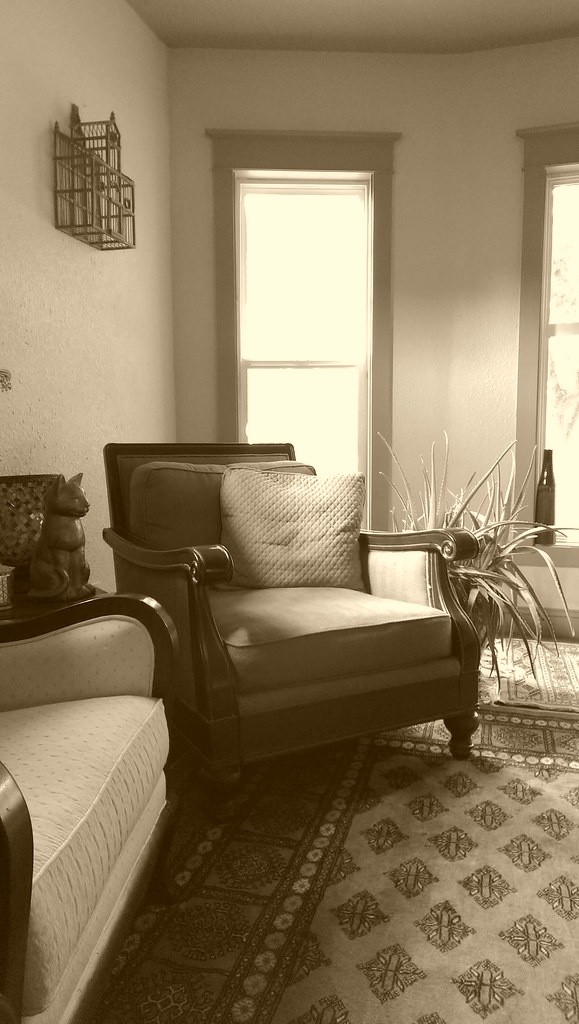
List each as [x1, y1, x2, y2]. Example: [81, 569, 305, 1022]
[534, 449, 556, 545]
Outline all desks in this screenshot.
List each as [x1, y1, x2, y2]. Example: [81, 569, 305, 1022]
[0, 583, 108, 621]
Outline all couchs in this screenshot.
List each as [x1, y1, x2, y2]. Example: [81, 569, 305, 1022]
[101, 442, 483, 818]
[1, 603, 183, 1024]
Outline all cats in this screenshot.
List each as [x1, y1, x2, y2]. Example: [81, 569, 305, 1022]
[27, 472, 96, 603]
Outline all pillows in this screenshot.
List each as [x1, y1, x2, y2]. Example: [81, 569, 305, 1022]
[130, 461, 317, 544]
[214, 468, 370, 593]
